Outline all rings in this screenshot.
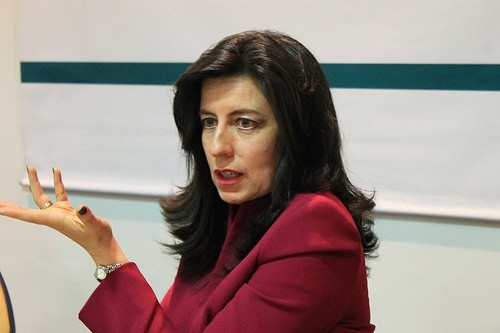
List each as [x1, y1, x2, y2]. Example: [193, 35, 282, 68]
[39, 200, 52, 210]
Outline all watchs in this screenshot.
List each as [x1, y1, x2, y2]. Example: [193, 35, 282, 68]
[94, 263, 125, 283]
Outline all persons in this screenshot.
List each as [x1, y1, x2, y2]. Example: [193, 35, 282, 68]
[0, 30, 380, 333]
